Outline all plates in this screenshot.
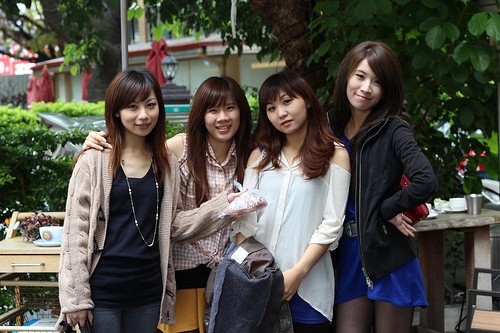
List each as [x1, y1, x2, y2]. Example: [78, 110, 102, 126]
[443, 208, 467, 212]
[427, 214, 438, 218]
[33, 239, 61, 246]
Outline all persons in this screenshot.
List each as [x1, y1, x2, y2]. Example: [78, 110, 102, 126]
[322, 40, 439, 333]
[57, 66, 181, 333]
[228, 70, 351, 333]
[79, 75, 254, 333]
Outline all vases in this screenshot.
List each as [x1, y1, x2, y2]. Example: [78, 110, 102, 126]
[463, 193, 482, 215]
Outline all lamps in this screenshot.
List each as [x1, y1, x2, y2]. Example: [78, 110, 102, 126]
[160, 52, 179, 87]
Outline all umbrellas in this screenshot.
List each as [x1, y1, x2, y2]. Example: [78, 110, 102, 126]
[145, 38, 169, 87]
[37, 64, 55, 103]
[27, 73, 38, 110]
[82, 64, 93, 102]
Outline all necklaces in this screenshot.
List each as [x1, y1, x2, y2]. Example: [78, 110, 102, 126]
[118, 151, 159, 246]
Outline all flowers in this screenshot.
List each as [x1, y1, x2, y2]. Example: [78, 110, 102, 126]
[456, 147, 487, 193]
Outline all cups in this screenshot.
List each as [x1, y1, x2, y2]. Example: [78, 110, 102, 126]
[427, 204, 431, 212]
[449, 198, 466, 209]
[464, 193, 484, 215]
[434, 200, 449, 210]
[39, 226, 62, 242]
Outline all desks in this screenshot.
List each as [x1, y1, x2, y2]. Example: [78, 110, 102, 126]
[0, 236, 62, 333]
[414, 208, 500, 333]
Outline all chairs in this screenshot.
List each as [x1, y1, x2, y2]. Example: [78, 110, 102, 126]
[468, 266, 500, 333]
